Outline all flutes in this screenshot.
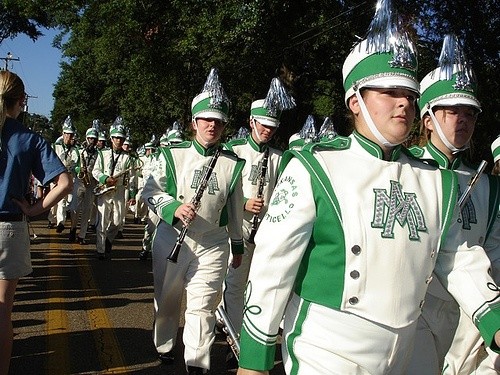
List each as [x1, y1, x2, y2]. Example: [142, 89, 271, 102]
[247, 148, 269, 243]
[165, 149, 221, 264]
[458, 160, 488, 208]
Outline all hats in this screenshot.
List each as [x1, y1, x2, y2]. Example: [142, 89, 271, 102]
[491, 136, 500, 163]
[63, 116, 75, 134]
[167, 121, 184, 142]
[110, 116, 127, 139]
[145, 135, 156, 149]
[160, 133, 168, 146]
[123, 137, 130, 145]
[97, 131, 106, 140]
[417, 32, 483, 120]
[288, 115, 316, 151]
[250, 77, 297, 128]
[86, 120, 98, 139]
[343, 1, 421, 108]
[190, 67, 231, 129]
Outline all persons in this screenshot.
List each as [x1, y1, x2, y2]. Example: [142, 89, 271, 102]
[27, 0, 500, 375]
[0, 70, 73, 375]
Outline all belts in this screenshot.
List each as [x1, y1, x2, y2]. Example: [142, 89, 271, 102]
[1, 212, 24, 222]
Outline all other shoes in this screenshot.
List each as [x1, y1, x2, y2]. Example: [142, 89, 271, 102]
[70, 228, 77, 242]
[97, 253, 105, 260]
[115, 230, 123, 239]
[159, 351, 176, 361]
[138, 248, 150, 261]
[187, 366, 204, 375]
[106, 237, 112, 253]
[48, 222, 54, 229]
[55, 222, 65, 234]
[78, 237, 87, 245]
[134, 217, 139, 223]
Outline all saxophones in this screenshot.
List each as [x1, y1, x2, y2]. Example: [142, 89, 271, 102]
[77, 146, 92, 188]
[93, 168, 129, 196]
